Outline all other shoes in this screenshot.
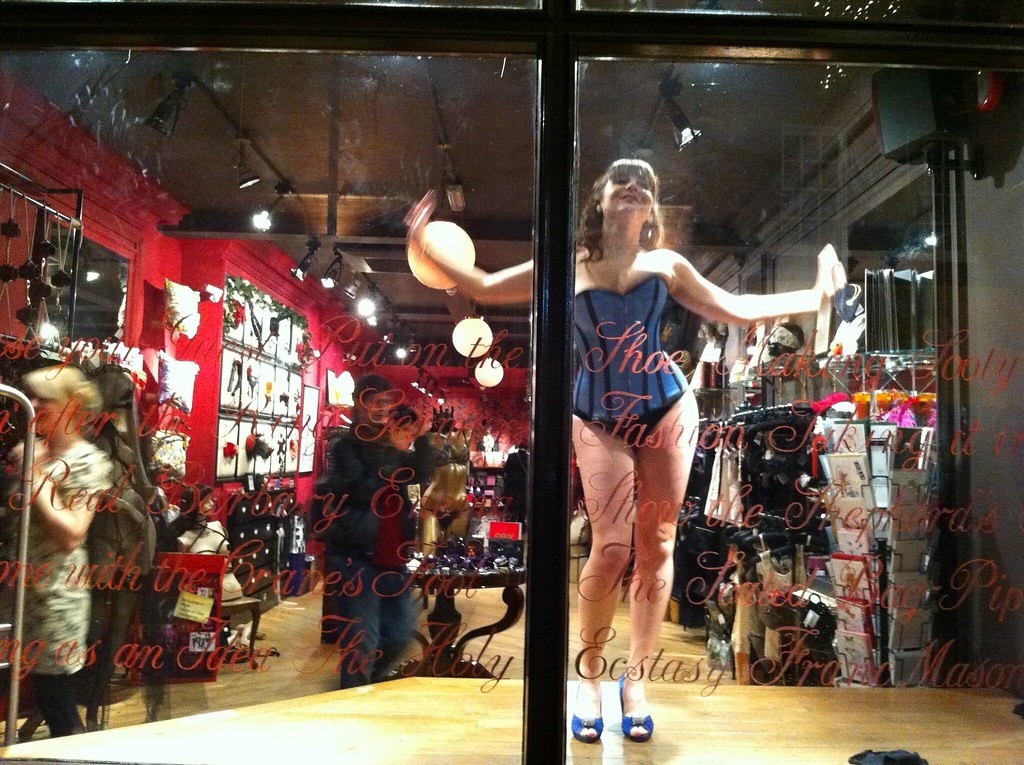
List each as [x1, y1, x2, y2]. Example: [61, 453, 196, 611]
[619, 674, 653, 741]
[571, 684, 604, 743]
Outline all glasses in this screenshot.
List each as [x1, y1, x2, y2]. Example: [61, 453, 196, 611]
[611, 173, 652, 190]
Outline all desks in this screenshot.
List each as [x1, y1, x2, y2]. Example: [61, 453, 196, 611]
[397, 564, 527, 678]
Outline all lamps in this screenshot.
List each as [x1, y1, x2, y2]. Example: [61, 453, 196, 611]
[233, 138, 261, 189]
[475, 358, 504, 387]
[408, 220, 476, 290]
[452, 318, 493, 358]
[291, 242, 324, 281]
[321, 255, 344, 288]
[658, 81, 704, 149]
[342, 279, 362, 299]
[446, 184, 466, 211]
[142, 84, 189, 134]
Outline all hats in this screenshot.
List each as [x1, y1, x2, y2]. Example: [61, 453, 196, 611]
[352, 375, 392, 401]
[388, 404, 417, 426]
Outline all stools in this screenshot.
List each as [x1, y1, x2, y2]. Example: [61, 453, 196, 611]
[221, 596, 261, 669]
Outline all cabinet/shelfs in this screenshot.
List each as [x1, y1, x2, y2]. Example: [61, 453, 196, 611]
[230, 488, 297, 614]
[814, 349, 940, 685]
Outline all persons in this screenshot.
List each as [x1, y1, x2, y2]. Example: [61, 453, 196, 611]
[9, 365, 112, 736]
[505, 438, 529, 533]
[311, 374, 434, 690]
[407, 158, 836, 743]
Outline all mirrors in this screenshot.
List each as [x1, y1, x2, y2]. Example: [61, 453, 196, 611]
[30, 216, 130, 363]
[838, 170, 936, 354]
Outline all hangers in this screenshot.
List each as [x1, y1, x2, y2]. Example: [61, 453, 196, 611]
[714, 407, 815, 438]
[686, 496, 830, 567]
